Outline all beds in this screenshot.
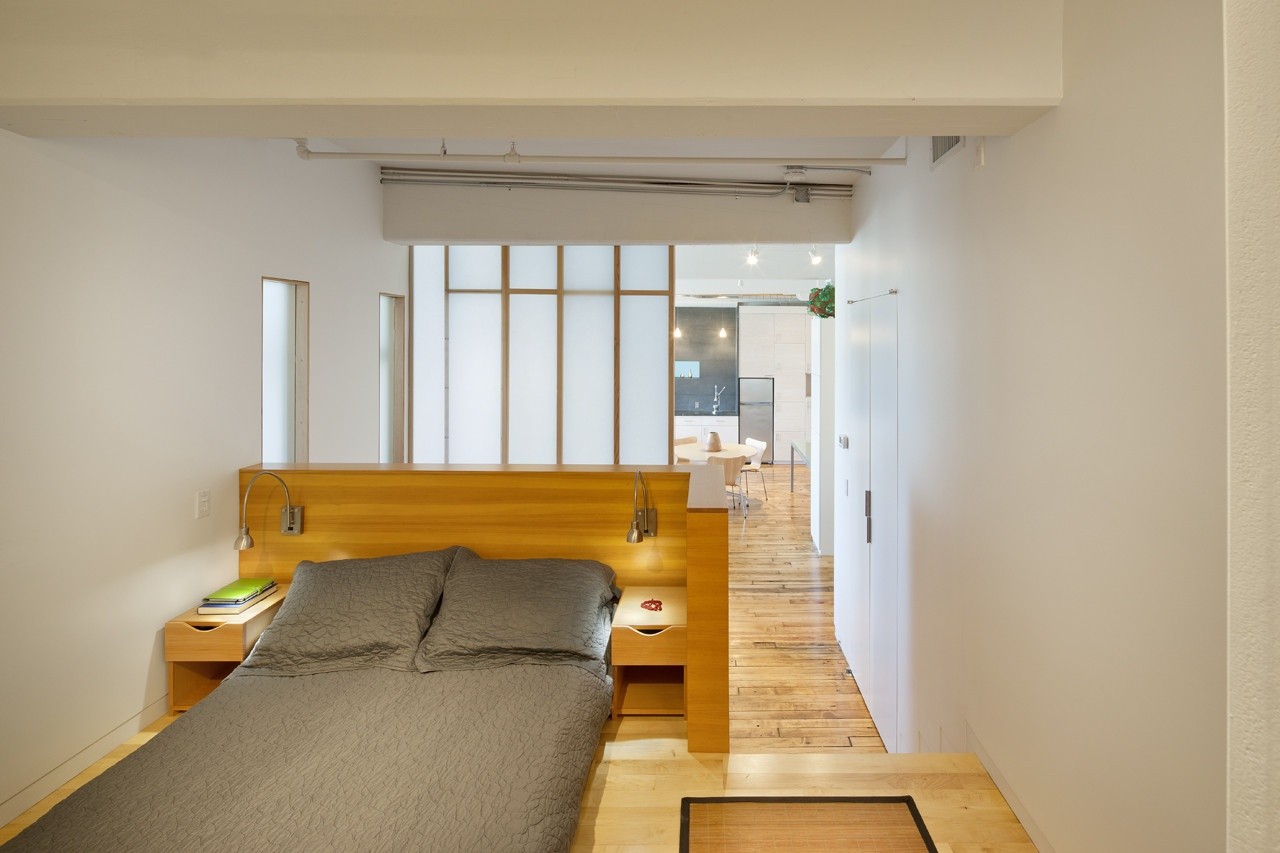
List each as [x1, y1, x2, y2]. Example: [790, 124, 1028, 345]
[1, 460, 729, 853]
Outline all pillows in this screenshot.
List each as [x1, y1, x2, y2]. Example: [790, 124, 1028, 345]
[412, 544, 618, 683]
[236, 544, 461, 676]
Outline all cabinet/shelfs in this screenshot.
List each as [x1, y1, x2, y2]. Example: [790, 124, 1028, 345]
[674, 410, 740, 444]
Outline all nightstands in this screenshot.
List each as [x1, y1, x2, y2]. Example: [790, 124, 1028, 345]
[164, 582, 291, 718]
[608, 582, 689, 722]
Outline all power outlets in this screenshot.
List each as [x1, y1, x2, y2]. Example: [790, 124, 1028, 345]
[695, 401, 700, 408]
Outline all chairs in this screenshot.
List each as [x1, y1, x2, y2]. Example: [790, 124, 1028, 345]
[673, 435, 697, 463]
[736, 438, 768, 505]
[708, 455, 748, 519]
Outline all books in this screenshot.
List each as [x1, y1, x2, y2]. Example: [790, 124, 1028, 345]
[197, 581, 279, 616]
[202, 577, 275, 605]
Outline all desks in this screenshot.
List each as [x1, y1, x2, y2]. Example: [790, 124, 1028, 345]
[676, 441, 757, 505]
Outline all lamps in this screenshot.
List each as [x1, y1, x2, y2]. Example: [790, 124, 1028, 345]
[719, 301, 728, 338]
[745, 244, 759, 264]
[232, 471, 305, 547]
[673, 296, 681, 338]
[808, 244, 822, 265]
[625, 468, 658, 544]
